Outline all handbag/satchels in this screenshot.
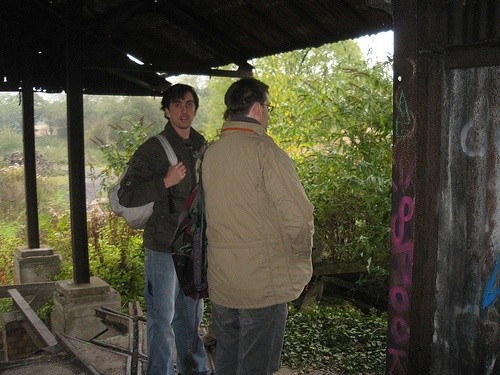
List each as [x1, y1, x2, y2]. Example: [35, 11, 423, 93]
[108, 133, 179, 229]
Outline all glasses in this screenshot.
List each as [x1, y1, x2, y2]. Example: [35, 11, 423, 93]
[259, 103, 274, 112]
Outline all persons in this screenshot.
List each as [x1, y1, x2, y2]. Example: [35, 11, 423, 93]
[202, 76, 314, 375]
[117, 83, 208, 375]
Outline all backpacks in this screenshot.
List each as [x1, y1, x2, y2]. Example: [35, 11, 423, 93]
[170, 141, 218, 300]
